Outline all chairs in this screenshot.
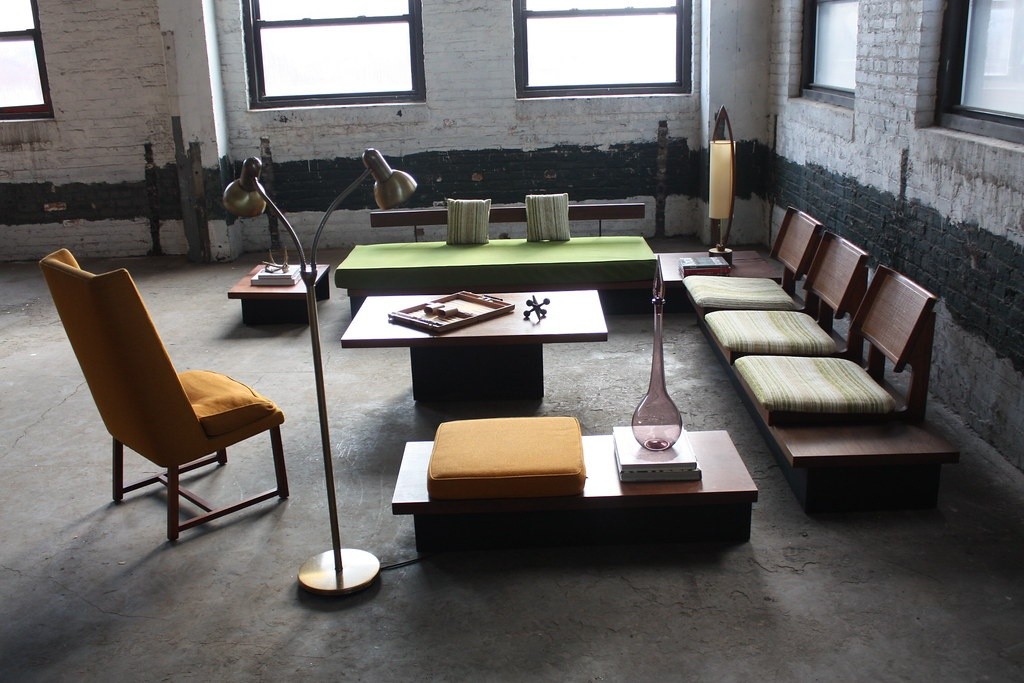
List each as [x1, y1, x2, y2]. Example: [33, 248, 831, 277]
[37, 247, 289, 542]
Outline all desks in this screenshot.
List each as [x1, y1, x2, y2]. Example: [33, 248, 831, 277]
[341, 291, 608, 405]
[657, 251, 783, 314]
[392, 429, 755, 554]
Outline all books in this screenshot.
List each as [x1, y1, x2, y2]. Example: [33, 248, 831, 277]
[678, 256, 730, 278]
[612, 425, 702, 483]
[251, 265, 301, 285]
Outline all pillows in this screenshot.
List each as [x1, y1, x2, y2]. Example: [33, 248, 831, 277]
[446, 199, 492, 244]
[525, 193, 570, 242]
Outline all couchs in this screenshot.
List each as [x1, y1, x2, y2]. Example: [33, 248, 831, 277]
[334, 202, 658, 320]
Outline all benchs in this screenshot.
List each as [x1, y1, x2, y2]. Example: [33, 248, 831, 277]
[682, 206, 961, 513]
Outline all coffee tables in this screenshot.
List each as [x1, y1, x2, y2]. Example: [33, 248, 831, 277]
[228, 264, 331, 326]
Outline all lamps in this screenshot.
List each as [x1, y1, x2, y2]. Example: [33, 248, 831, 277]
[222, 150, 418, 599]
[708, 104, 736, 265]
